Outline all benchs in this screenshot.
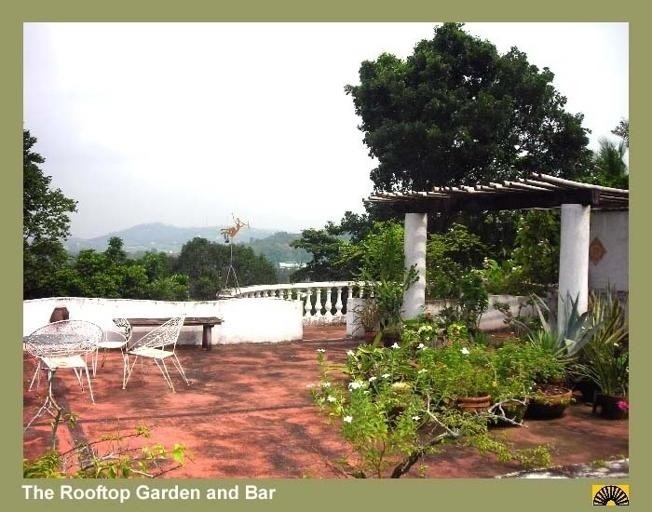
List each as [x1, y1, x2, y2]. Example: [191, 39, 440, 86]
[111, 315, 224, 353]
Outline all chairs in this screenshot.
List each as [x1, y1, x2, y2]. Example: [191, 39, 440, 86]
[23, 305, 192, 411]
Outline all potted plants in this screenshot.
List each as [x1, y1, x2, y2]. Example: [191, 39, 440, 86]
[343, 267, 628, 432]
